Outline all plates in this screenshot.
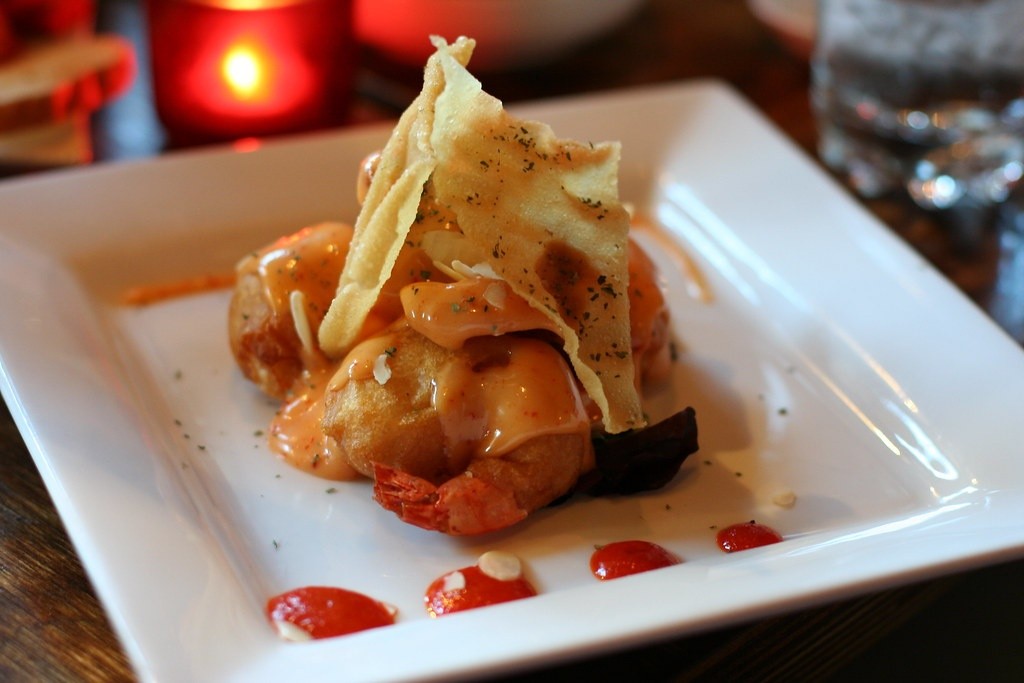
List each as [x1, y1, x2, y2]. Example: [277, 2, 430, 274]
[0, 76, 1024, 683]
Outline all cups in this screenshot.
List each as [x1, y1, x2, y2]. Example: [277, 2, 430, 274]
[146, 1, 355, 148]
[811, 0, 1024, 211]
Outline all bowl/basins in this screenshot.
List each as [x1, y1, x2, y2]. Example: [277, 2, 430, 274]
[350, 0, 635, 72]
